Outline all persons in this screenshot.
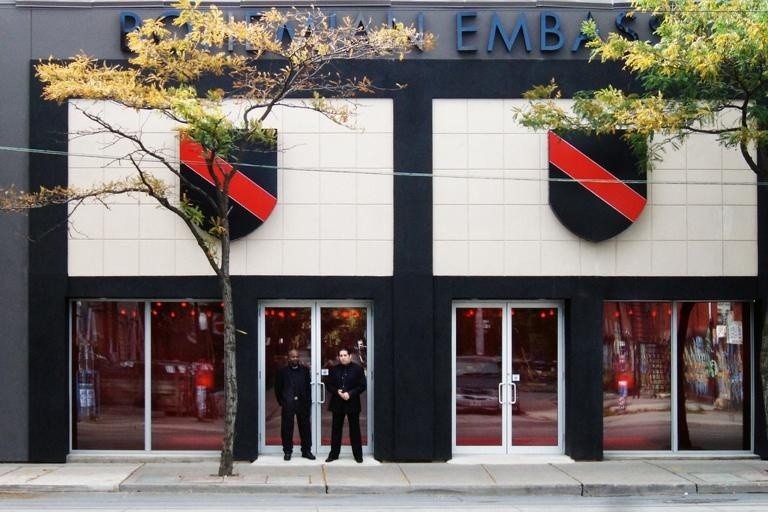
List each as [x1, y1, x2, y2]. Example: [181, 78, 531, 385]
[274, 347, 318, 461]
[323, 345, 368, 463]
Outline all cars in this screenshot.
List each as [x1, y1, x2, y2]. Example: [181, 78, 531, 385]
[272, 335, 325, 368]
[100, 360, 211, 415]
[456, 355, 519, 415]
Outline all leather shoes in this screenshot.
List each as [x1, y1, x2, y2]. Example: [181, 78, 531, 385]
[325, 453, 339, 462]
[283, 452, 291, 460]
[301, 451, 315, 459]
[353, 454, 363, 462]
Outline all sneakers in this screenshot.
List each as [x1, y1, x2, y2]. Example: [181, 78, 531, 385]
[618, 395, 640, 409]
